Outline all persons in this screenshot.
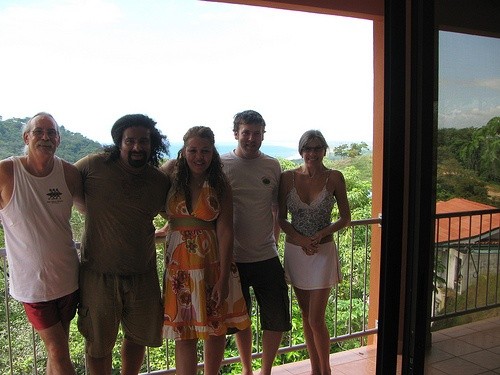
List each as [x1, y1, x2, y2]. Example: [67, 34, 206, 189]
[219, 110, 293, 375]
[276, 130, 351, 375]
[158, 126, 253, 375]
[0, 113, 86, 375]
[70, 114, 170, 375]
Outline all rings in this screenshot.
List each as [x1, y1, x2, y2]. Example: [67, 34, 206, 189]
[309, 249, 312, 251]
